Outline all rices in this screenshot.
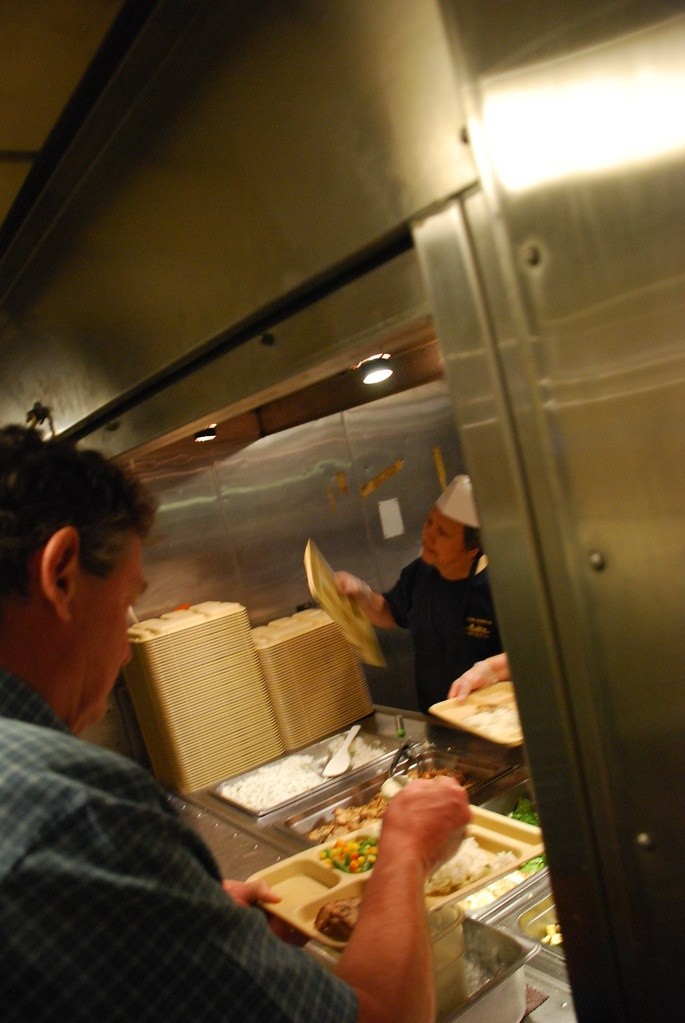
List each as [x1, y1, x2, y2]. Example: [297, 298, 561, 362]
[462, 707, 524, 742]
[425, 836, 518, 896]
[219, 736, 386, 811]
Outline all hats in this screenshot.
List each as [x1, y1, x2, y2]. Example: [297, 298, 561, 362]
[436, 473, 481, 531]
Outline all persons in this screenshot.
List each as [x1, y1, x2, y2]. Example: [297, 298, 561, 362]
[446, 651, 510, 701]
[333, 476, 499, 715]
[1, 443, 470, 1022]
